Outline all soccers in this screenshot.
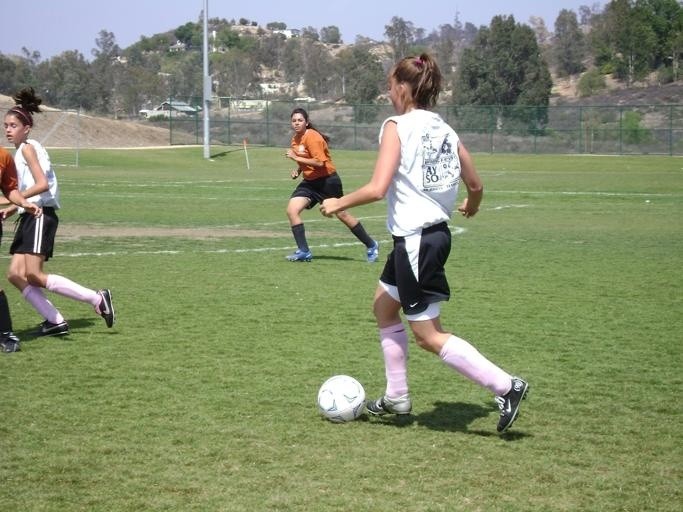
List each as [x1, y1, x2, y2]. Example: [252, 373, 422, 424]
[318, 375, 365, 423]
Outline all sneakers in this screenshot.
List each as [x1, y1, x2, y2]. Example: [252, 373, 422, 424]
[25, 320, 70, 337]
[365, 393, 412, 418]
[95, 288, 116, 329]
[367, 241, 379, 262]
[0, 332, 21, 353]
[496, 376, 530, 432]
[285, 250, 313, 261]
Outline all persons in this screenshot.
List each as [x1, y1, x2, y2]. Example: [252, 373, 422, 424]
[0, 146, 44, 355]
[284, 107, 378, 262]
[0, 87, 114, 336]
[318, 52, 528, 435]
